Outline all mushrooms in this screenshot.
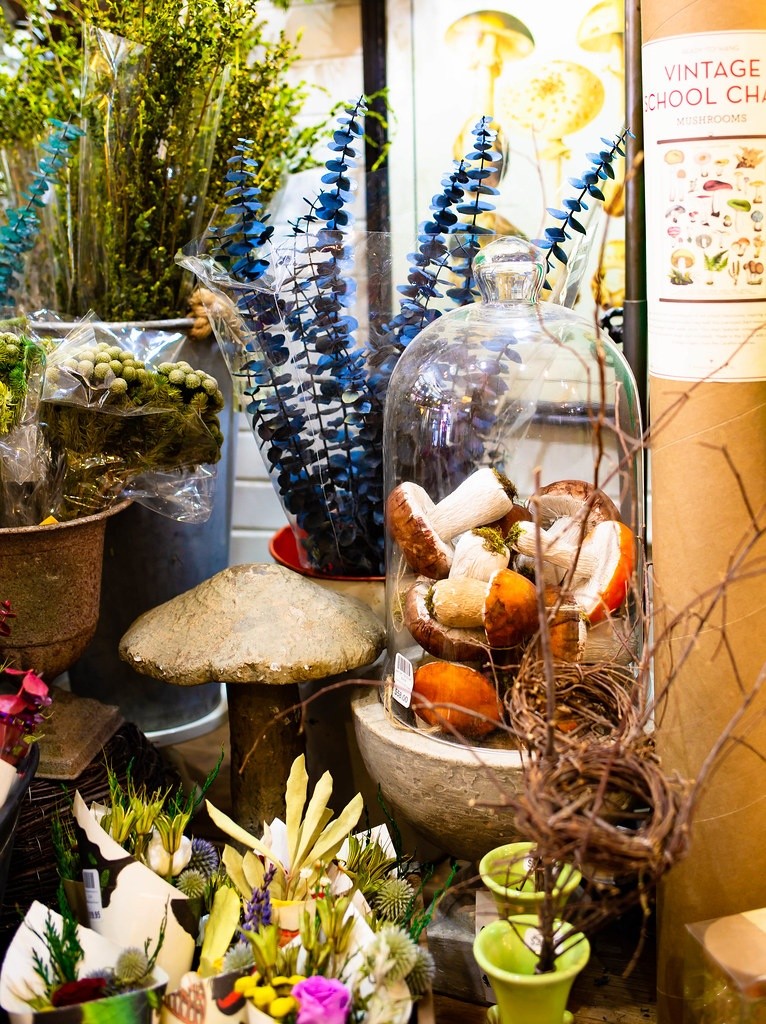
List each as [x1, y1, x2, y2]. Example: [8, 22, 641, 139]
[389, 466, 642, 733]
[119, 563, 386, 843]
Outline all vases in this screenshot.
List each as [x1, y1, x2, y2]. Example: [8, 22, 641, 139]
[17, 308, 229, 748]
[0, 494, 136, 779]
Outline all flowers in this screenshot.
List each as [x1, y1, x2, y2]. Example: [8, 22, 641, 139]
[0, 742, 463, 1024]
[0, 0, 636, 575]
